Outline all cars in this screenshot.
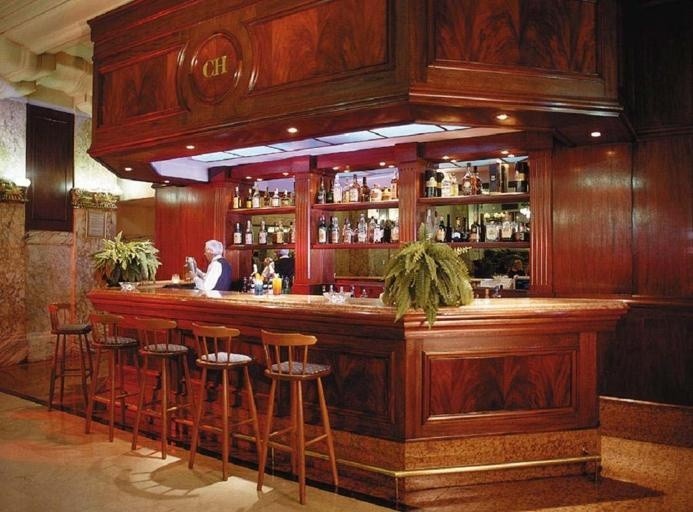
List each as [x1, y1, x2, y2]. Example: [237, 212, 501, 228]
[271, 278, 283, 295]
[254, 280, 264, 295]
[187, 257, 197, 275]
[171, 274, 180, 284]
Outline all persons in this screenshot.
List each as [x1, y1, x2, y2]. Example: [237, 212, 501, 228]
[185, 240, 232, 291]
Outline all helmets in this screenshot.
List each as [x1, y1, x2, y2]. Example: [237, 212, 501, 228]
[118, 282, 140, 291]
[323, 292, 352, 305]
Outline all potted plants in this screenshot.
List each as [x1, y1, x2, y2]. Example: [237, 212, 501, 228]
[89, 230, 163, 288]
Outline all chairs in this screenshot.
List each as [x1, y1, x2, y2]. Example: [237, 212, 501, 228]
[131, 315, 202, 460]
[48, 302, 96, 412]
[83, 313, 148, 443]
[187, 322, 261, 482]
[256, 329, 339, 504]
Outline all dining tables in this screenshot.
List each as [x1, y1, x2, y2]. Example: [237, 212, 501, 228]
[89, 230, 163, 288]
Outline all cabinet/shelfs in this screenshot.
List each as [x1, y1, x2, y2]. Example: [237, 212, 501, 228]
[315, 172, 399, 204]
[317, 213, 399, 244]
[258, 220, 267, 245]
[233, 222, 243, 245]
[425, 161, 529, 197]
[423, 208, 530, 243]
[271, 220, 296, 244]
[321, 284, 369, 298]
[207, 131, 544, 297]
[246, 186, 295, 209]
[232, 187, 241, 209]
[245, 220, 254, 244]
[249, 284, 256, 294]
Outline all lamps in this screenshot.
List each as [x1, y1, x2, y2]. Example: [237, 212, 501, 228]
[187, 322, 261, 482]
[131, 315, 202, 460]
[83, 313, 148, 443]
[48, 302, 96, 412]
[256, 329, 339, 504]
